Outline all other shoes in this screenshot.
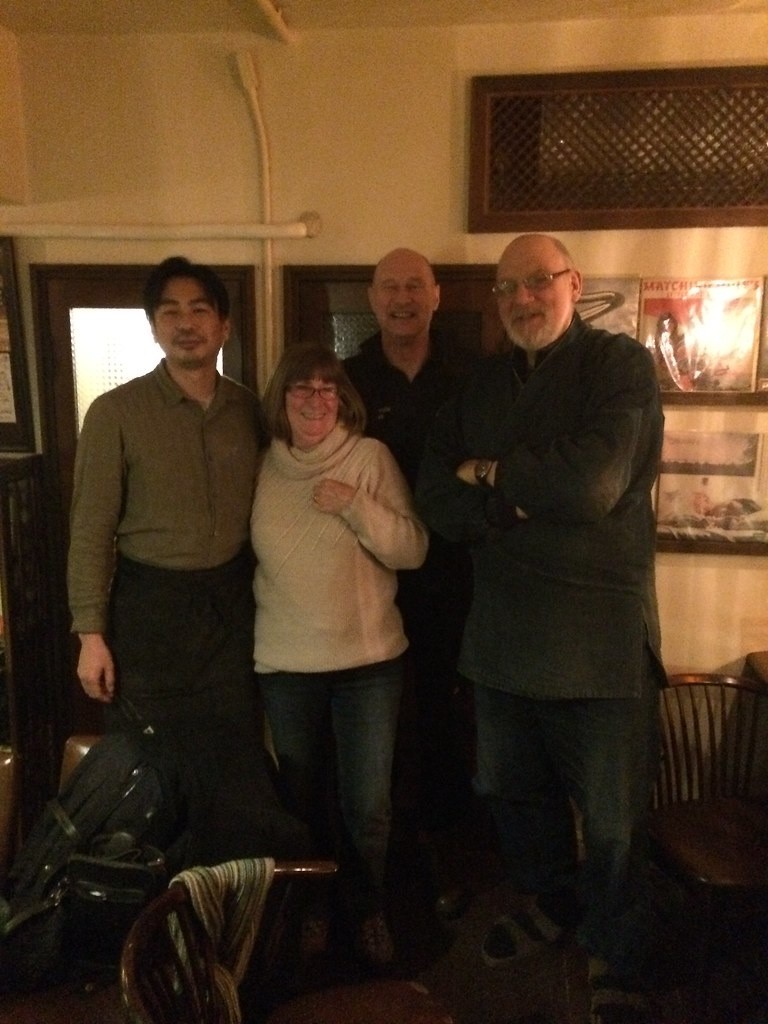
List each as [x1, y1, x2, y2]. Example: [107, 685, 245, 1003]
[356, 907, 394, 976]
[300, 895, 334, 953]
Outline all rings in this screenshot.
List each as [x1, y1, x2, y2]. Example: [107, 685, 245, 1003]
[314, 496, 317, 501]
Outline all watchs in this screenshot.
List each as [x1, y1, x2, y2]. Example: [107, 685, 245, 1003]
[475, 459, 493, 486]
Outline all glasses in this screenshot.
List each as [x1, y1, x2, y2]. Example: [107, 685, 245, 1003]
[284, 384, 339, 401]
[491, 268, 571, 298]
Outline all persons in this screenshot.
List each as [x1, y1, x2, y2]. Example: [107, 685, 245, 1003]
[248, 343, 430, 981]
[414, 234, 665, 1024]
[67, 256, 269, 863]
[344, 248, 468, 780]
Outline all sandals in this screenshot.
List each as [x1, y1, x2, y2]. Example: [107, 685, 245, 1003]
[587, 950, 648, 1024]
[481, 895, 578, 968]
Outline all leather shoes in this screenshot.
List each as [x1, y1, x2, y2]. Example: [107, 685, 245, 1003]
[436, 865, 475, 917]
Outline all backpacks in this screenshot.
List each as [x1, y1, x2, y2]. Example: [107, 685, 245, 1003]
[0, 730, 173, 997]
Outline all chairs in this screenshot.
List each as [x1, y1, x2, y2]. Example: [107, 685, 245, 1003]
[655, 673, 767, 928]
[117, 859, 453, 1024]
